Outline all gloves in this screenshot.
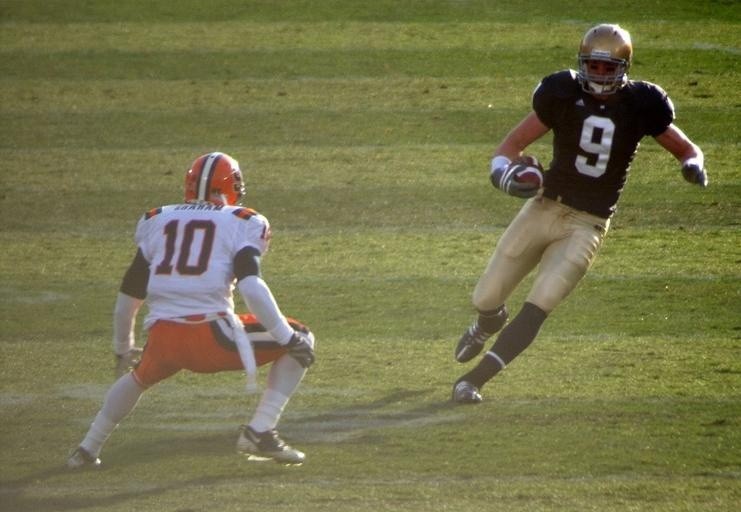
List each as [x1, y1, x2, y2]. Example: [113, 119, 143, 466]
[282, 331, 315, 369]
[491, 162, 538, 198]
[114, 347, 144, 378]
[681, 163, 708, 187]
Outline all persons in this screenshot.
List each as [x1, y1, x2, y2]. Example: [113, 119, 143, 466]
[448, 23, 710, 404]
[65, 150, 319, 474]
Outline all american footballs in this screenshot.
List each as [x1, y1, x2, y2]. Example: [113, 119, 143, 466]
[510, 156, 545, 190]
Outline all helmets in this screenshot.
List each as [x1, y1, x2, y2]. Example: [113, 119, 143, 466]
[184, 152, 247, 207]
[576, 23, 633, 97]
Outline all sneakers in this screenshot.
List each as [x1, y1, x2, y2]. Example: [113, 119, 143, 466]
[454, 306, 509, 363]
[236, 425, 305, 465]
[452, 381, 483, 403]
[65, 448, 102, 473]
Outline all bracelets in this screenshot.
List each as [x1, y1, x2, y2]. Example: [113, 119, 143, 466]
[491, 155, 513, 174]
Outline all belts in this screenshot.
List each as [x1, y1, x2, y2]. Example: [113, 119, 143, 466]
[543, 190, 610, 219]
